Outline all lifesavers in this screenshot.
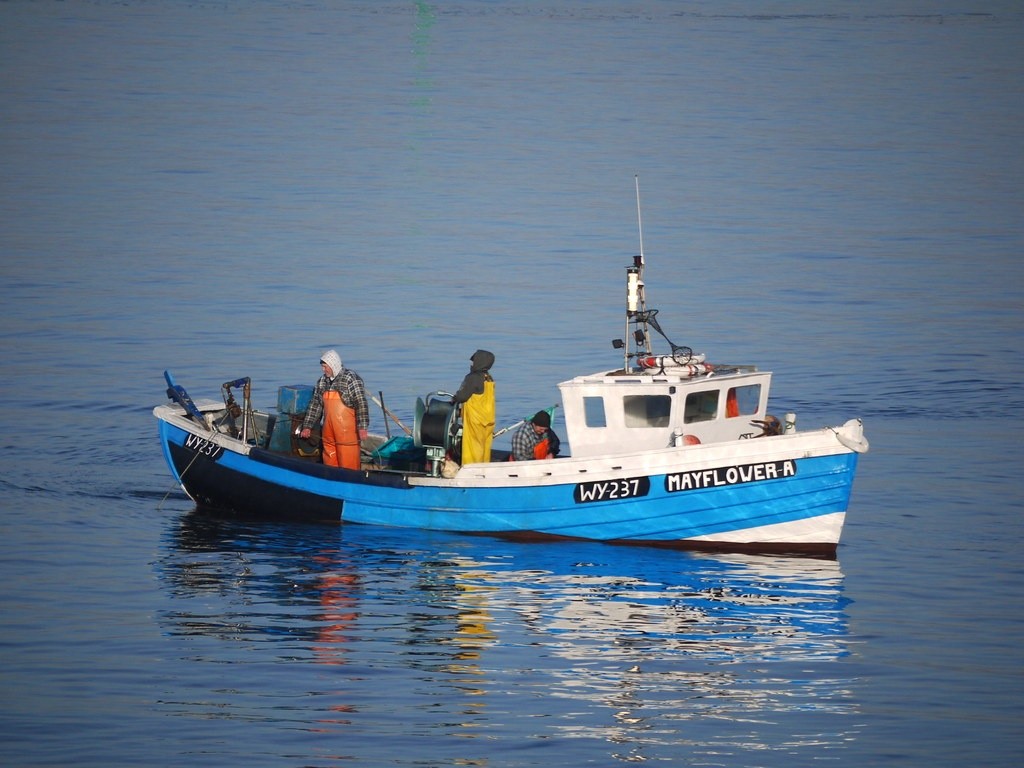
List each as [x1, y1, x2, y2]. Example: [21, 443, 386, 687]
[644, 363, 710, 377]
[636, 352, 705, 367]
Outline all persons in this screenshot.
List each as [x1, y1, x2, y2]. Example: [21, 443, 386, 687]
[448, 348, 503, 474]
[296, 348, 374, 473]
[506, 408, 564, 469]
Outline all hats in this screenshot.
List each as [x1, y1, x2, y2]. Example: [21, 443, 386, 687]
[469, 352, 474, 361]
[533, 410, 550, 427]
[319, 359, 326, 364]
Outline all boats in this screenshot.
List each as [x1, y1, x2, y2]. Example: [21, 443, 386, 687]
[151, 173, 870, 554]
